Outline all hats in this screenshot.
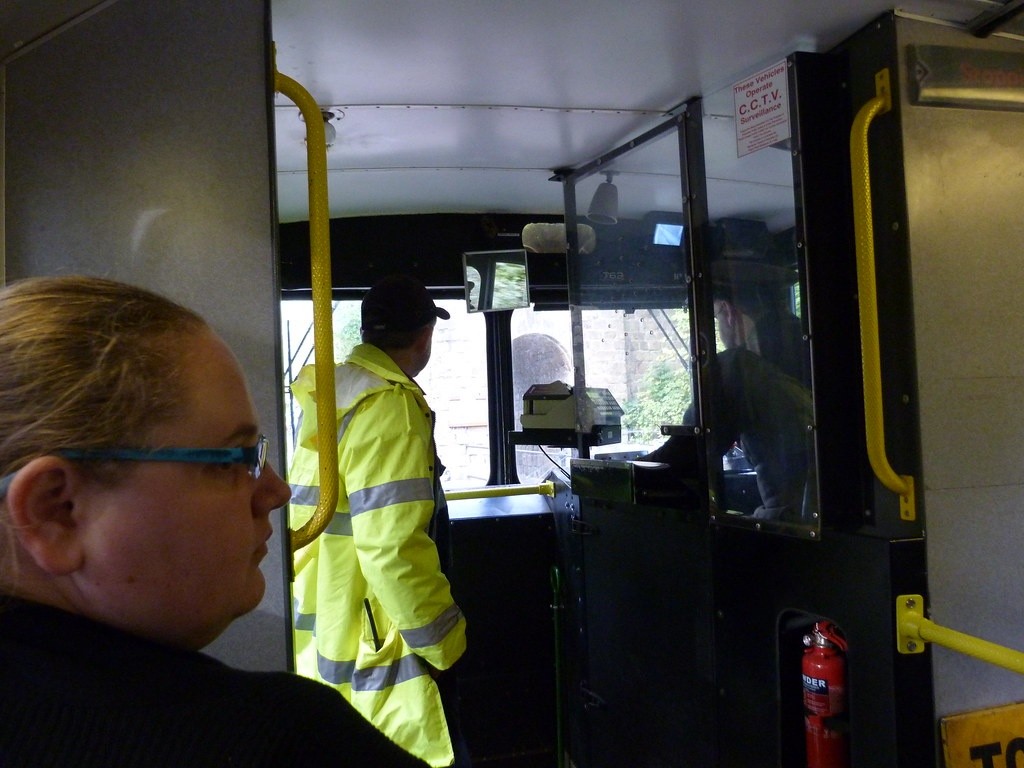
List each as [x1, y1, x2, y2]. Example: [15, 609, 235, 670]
[355, 276, 453, 339]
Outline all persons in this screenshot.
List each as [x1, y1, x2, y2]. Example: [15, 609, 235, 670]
[1, 273, 431, 767]
[289, 276, 466, 768]
[627, 251, 819, 528]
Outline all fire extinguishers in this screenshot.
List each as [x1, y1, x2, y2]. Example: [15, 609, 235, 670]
[801, 618, 849, 767]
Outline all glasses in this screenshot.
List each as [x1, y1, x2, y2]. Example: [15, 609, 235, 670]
[18, 419, 271, 482]
